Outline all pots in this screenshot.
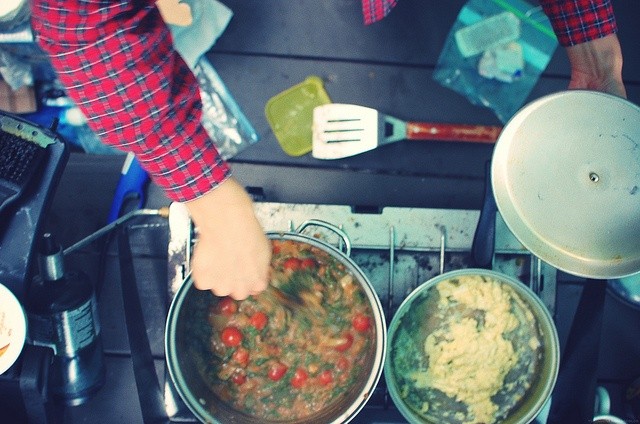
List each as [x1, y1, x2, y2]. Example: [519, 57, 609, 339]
[385, 268, 561, 424]
[163, 219, 386, 423]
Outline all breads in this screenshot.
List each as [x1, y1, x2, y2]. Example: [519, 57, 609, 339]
[0, 311, 13, 355]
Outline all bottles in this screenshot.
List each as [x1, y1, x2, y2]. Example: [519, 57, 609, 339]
[25, 231, 105, 405]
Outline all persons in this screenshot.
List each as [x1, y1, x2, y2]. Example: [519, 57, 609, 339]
[25, 0, 628, 301]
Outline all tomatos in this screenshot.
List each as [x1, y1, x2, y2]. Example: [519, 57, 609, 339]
[229, 347, 249, 366]
[334, 332, 354, 354]
[291, 370, 308, 388]
[352, 312, 370, 334]
[221, 325, 243, 349]
[318, 368, 332, 387]
[250, 309, 267, 332]
[232, 374, 245, 386]
[301, 258, 316, 273]
[216, 297, 237, 318]
[267, 360, 287, 381]
[280, 257, 300, 274]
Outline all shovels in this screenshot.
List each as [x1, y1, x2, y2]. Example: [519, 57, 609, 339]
[312, 103, 503, 160]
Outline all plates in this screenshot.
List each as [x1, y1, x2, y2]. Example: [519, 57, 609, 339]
[0, 282, 28, 376]
